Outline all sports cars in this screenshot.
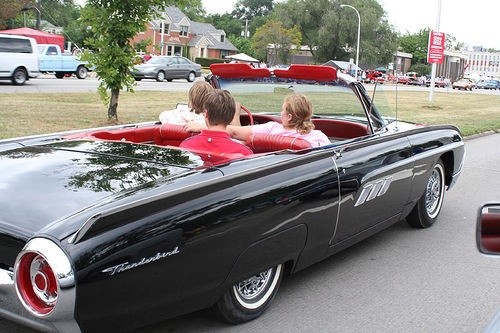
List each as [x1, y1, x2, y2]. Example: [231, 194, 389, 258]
[1, 60, 465, 333]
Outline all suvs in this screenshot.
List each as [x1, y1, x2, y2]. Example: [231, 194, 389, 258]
[362, 70, 386, 86]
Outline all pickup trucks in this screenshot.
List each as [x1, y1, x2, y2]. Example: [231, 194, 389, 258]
[37, 43, 94, 81]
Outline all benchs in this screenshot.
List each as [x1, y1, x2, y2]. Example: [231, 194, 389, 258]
[89, 123, 313, 166]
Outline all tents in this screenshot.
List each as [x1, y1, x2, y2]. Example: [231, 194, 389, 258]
[0, 27, 64, 54]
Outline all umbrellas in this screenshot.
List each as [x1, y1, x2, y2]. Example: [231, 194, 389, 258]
[374, 66, 387, 73]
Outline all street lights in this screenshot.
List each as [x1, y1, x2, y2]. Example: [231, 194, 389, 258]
[340, 4, 361, 79]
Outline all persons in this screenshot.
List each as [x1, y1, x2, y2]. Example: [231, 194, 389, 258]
[159, 73, 332, 155]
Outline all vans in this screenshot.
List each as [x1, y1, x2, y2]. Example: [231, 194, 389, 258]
[0, 33, 37, 83]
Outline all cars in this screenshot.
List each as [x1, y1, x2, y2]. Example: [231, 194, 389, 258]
[131, 56, 203, 82]
[395, 67, 500, 91]
